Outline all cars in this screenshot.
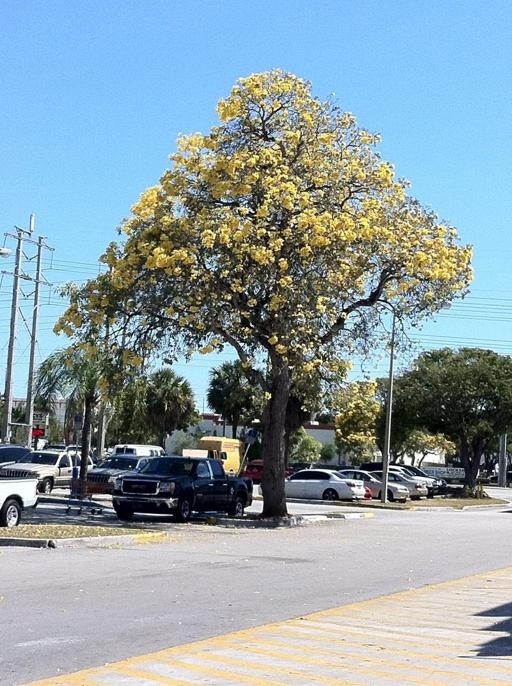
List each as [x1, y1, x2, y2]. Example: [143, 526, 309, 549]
[243, 459, 448, 500]
[490, 471, 512, 487]
[1, 444, 166, 493]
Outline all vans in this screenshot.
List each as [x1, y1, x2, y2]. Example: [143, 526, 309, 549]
[199, 436, 247, 477]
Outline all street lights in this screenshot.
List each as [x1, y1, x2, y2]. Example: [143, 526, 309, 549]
[380, 300, 452, 502]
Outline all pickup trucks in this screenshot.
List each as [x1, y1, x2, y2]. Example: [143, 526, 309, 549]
[112, 457, 253, 521]
[1, 470, 41, 527]
